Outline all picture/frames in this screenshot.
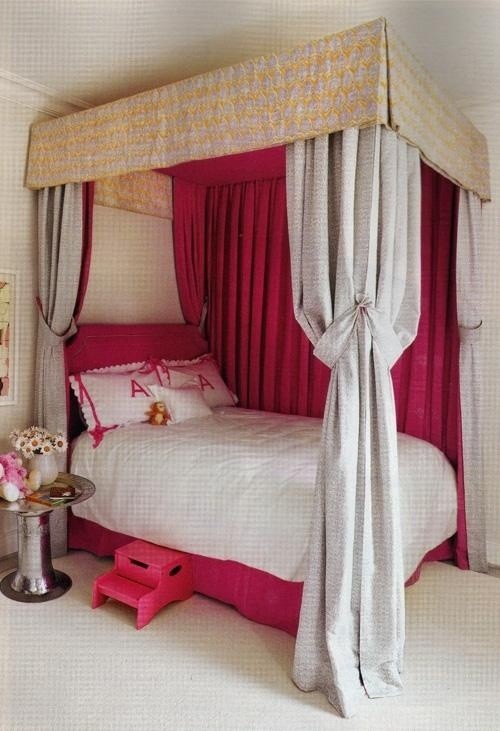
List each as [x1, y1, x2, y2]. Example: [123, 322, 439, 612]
[0, 269, 24, 408]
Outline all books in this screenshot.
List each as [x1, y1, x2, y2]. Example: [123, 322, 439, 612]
[49, 487, 75, 499]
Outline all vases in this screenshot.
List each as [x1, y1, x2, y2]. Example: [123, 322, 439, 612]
[24, 451, 59, 485]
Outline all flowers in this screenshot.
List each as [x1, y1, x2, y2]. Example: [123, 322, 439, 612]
[9, 424, 69, 460]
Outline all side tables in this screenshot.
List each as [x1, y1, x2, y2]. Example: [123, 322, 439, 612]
[2, 468, 91, 603]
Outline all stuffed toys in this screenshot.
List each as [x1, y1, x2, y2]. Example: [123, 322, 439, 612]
[0, 452, 41, 501]
[146, 402, 171, 426]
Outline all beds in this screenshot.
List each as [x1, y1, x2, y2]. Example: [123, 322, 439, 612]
[64, 321, 460, 640]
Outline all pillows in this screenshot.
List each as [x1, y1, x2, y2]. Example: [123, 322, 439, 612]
[69, 346, 232, 440]
